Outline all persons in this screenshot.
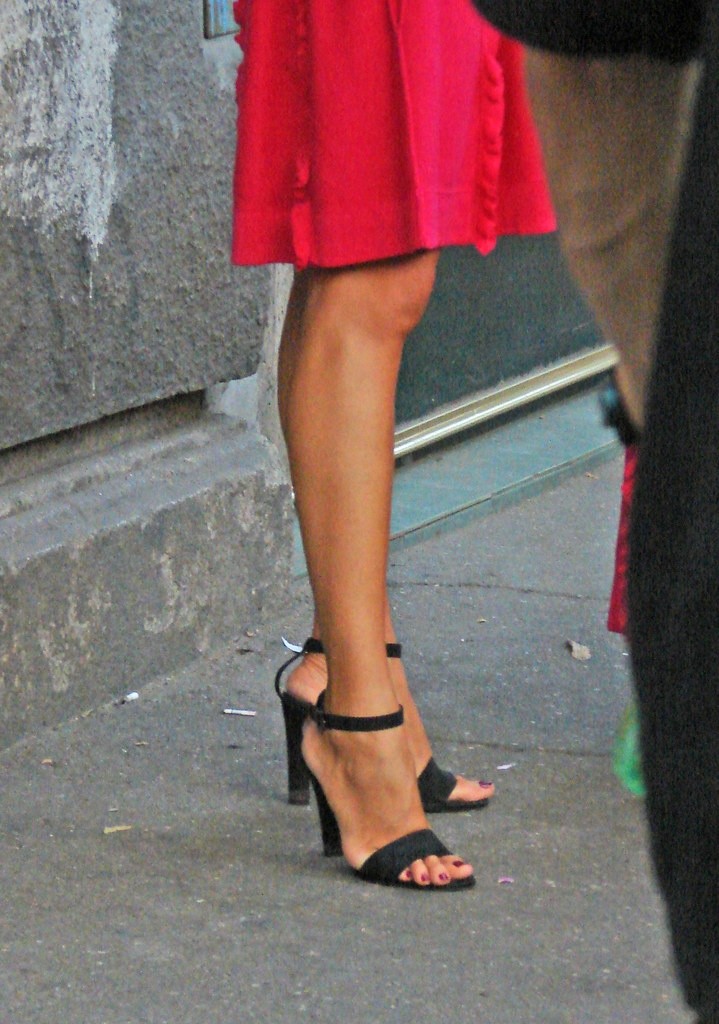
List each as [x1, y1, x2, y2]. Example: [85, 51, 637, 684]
[230, 0, 556, 893]
[475, 0, 719, 1024]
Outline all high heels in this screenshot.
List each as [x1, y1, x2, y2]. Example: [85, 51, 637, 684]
[299, 692, 475, 892]
[273, 637, 490, 813]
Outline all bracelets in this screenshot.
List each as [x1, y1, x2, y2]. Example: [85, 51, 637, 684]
[595, 371, 642, 449]
